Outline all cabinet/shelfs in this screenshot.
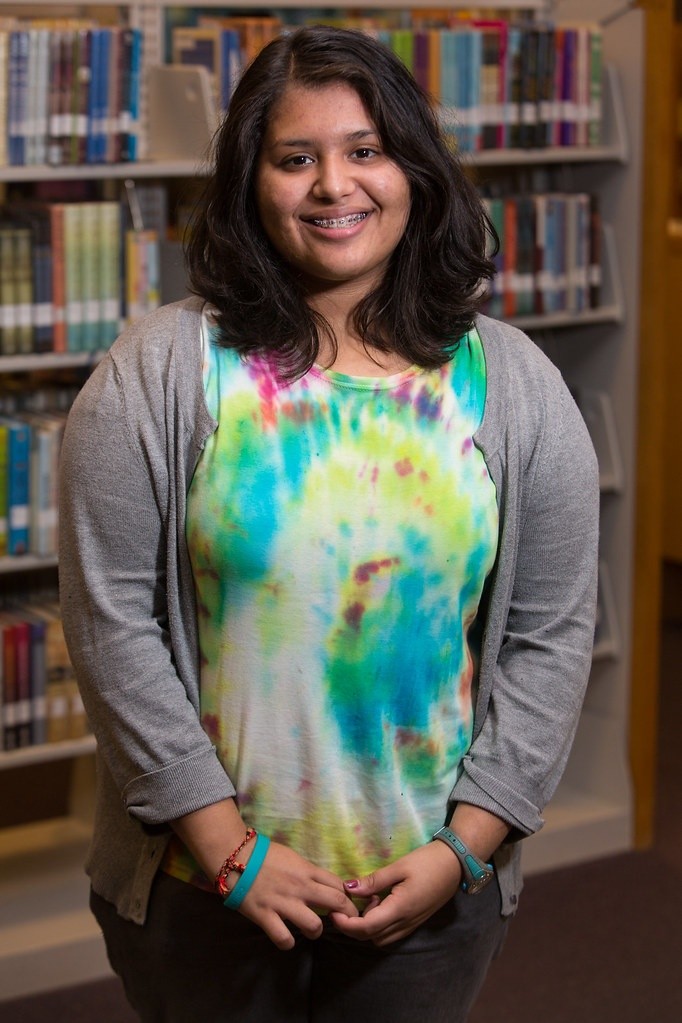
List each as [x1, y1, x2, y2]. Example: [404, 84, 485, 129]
[1, 0, 674, 1004]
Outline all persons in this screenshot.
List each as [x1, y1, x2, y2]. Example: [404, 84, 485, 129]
[57, 26, 601, 1023]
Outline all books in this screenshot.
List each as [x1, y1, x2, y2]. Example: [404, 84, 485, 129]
[1, 184, 623, 357]
[0, 10, 602, 166]
[0, 588, 93, 750]
[0, 376, 78, 556]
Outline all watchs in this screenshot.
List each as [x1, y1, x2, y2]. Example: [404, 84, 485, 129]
[433, 826, 494, 896]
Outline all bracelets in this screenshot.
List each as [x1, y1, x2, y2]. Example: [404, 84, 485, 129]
[224, 832, 271, 912]
[215, 826, 258, 894]
